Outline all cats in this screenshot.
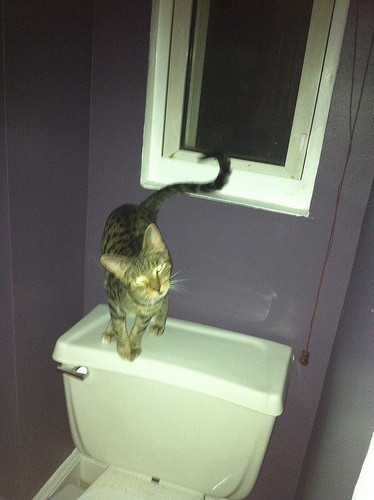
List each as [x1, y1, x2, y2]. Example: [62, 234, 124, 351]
[101, 148, 234, 361]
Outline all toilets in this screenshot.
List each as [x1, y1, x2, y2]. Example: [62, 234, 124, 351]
[26, 303, 294, 500]
